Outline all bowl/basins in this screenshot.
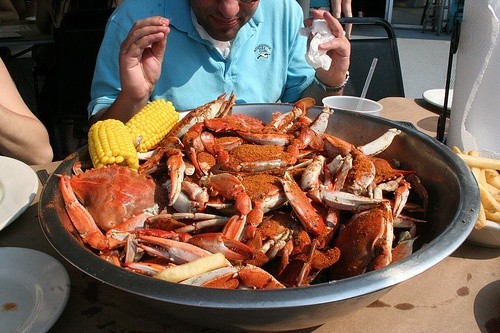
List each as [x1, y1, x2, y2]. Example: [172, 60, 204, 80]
[38, 104, 480, 333]
[467, 220, 500, 248]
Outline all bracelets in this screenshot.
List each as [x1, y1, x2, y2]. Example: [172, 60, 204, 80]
[314, 71, 350, 92]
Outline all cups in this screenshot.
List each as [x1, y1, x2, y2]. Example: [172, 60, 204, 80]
[321, 96, 383, 117]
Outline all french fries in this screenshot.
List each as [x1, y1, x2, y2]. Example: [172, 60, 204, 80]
[452, 146, 500, 230]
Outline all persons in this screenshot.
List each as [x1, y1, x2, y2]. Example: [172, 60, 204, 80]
[0, 57, 54, 165]
[87, 0, 351, 132]
[330, 0, 352, 40]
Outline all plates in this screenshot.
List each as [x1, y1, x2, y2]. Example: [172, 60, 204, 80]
[422, 89, 453, 109]
[0, 247, 69, 333]
[0, 156, 38, 230]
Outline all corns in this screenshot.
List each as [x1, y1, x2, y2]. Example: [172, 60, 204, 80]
[88, 118, 139, 172]
[125, 99, 181, 153]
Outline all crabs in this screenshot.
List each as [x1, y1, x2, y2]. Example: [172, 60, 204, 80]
[52, 90, 427, 288]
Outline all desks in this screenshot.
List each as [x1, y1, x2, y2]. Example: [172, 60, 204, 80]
[0, 98, 500, 333]
[0, 21, 55, 58]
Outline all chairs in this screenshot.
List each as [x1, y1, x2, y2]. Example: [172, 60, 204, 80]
[336, 17, 405, 101]
